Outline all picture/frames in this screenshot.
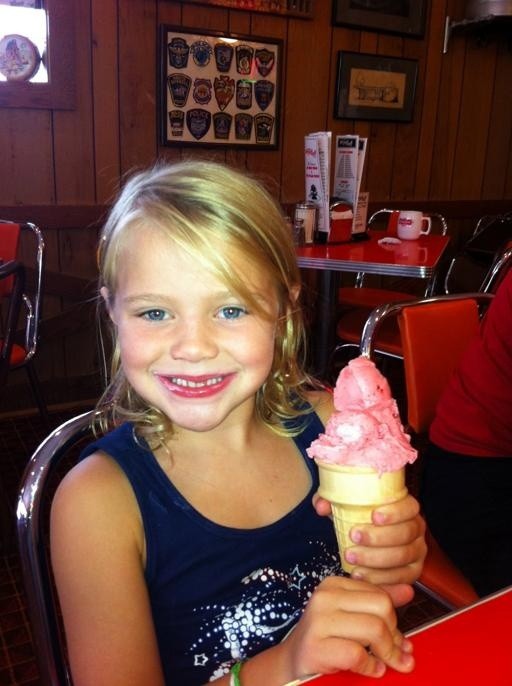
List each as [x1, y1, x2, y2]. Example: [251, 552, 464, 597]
[155, 23, 285, 152]
[330, 0, 427, 41]
[332, 49, 420, 125]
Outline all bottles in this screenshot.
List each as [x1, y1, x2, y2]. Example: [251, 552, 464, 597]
[295, 201, 317, 243]
[294, 218, 305, 246]
[285, 217, 293, 229]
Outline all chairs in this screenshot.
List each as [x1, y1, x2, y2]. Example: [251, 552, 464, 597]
[338, 208, 510, 370]
[360, 292, 494, 611]
[0, 220, 46, 372]
[17, 400, 131, 685]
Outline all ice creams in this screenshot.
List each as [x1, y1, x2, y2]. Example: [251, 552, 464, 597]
[305, 356, 419, 576]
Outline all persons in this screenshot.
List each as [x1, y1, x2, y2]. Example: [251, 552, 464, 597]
[420, 269, 512, 599]
[49, 152, 427, 686]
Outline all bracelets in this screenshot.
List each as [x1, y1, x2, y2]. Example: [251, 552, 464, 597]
[229, 662, 241, 686]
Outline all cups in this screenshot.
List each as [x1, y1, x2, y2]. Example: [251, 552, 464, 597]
[397, 210, 432, 240]
